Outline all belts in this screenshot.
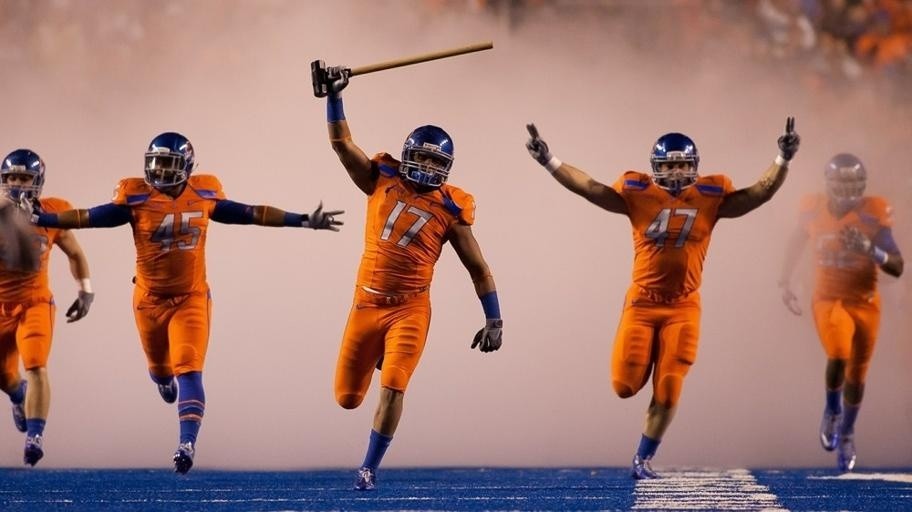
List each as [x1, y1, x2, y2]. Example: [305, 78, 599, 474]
[631, 287, 689, 306]
[1, 298, 44, 318]
[357, 286, 422, 309]
[137, 292, 190, 321]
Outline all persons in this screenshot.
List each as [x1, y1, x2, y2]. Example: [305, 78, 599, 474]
[327, 64, 503, 491]
[523, 117, 800, 480]
[757, 0, 912, 95]
[20, 132, 346, 475]
[778, 153, 904, 473]
[0, 148, 95, 467]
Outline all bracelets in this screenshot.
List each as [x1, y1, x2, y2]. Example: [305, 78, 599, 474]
[42, 212, 59, 227]
[479, 291, 501, 319]
[545, 156, 561, 174]
[327, 98, 345, 122]
[873, 243, 889, 267]
[76, 278, 93, 294]
[775, 155, 793, 170]
[284, 211, 301, 227]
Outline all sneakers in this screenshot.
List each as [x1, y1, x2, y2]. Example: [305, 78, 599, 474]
[818, 405, 859, 474]
[173, 440, 196, 474]
[632, 451, 661, 479]
[11, 379, 29, 433]
[157, 378, 177, 403]
[24, 432, 44, 466]
[354, 464, 376, 492]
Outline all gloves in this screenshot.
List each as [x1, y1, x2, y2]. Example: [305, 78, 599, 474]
[14, 191, 39, 227]
[309, 201, 345, 231]
[324, 65, 351, 93]
[526, 124, 553, 167]
[839, 225, 871, 257]
[776, 117, 799, 160]
[471, 318, 503, 352]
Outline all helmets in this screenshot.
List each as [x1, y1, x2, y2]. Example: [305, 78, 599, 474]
[650, 132, 700, 195]
[1, 149, 45, 204]
[824, 153, 868, 212]
[144, 132, 194, 192]
[398, 124, 455, 188]
